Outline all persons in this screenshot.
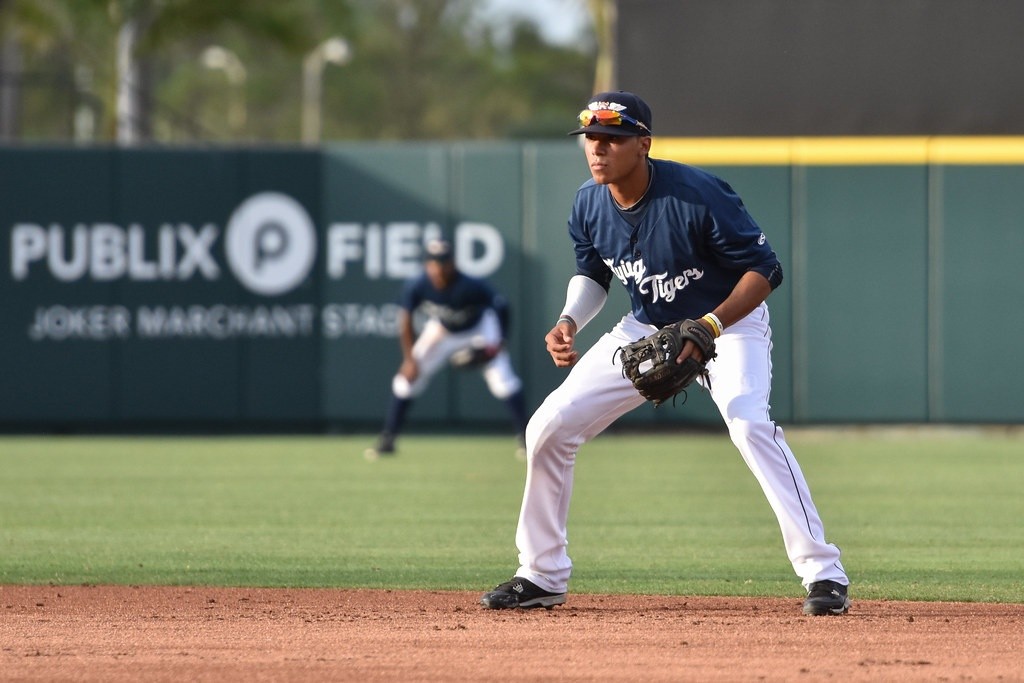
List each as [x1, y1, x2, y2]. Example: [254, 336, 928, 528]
[372, 239, 532, 458]
[482, 91, 852, 618]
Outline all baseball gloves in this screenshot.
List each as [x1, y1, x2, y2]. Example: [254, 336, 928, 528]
[620, 318, 718, 406]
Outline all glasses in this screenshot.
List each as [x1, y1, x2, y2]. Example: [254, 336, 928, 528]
[575, 109, 651, 134]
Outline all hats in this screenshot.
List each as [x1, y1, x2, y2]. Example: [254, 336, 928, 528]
[567, 90, 653, 137]
[421, 240, 452, 261]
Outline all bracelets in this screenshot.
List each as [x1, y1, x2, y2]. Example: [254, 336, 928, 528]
[702, 312, 723, 339]
[554, 317, 575, 328]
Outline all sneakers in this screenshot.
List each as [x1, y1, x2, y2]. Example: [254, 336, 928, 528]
[480, 576, 565, 608]
[802, 579, 848, 614]
